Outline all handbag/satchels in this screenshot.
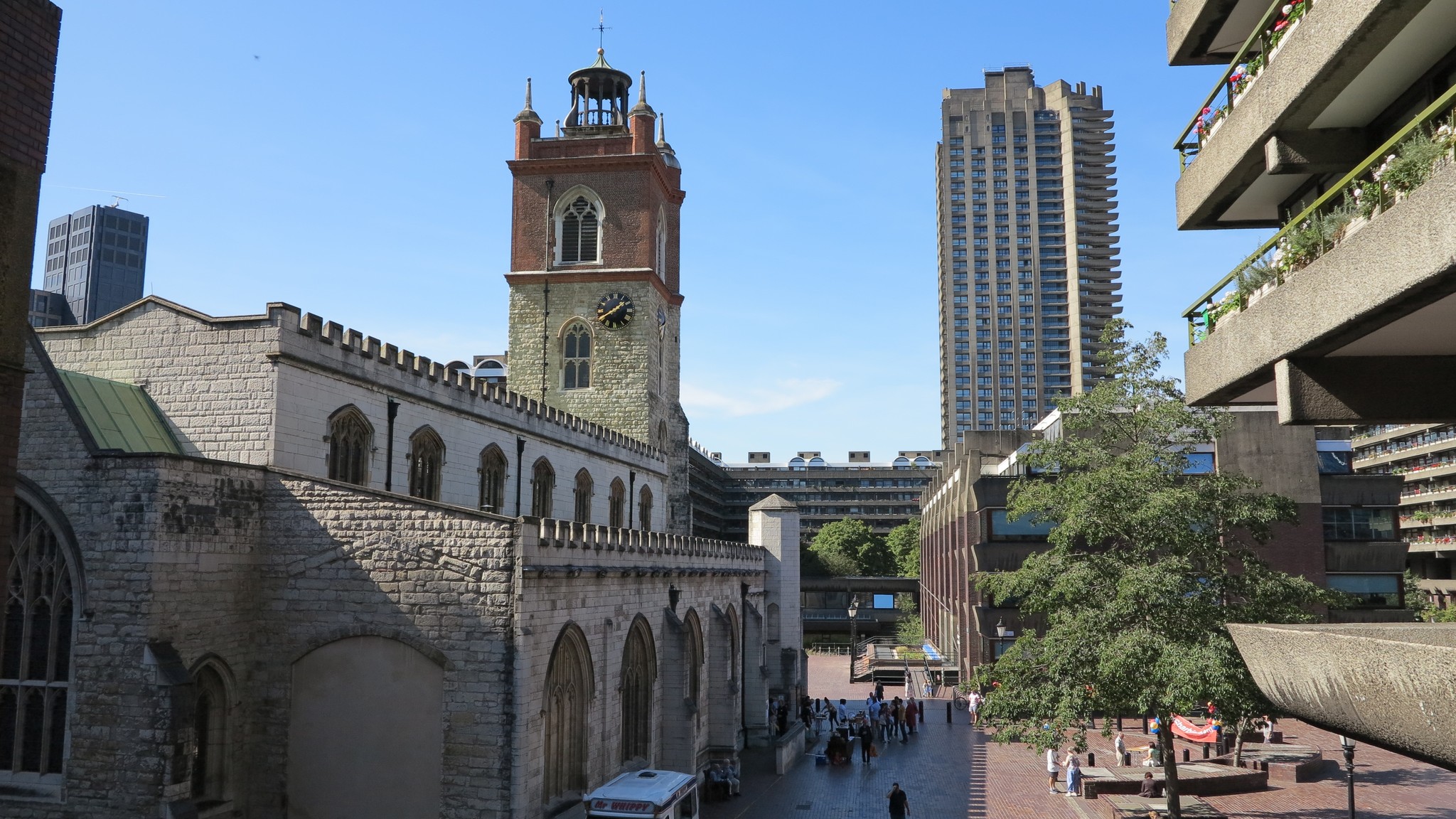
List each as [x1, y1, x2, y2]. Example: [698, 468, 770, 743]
[1142, 760, 1151, 767]
[805, 726, 815, 739]
[880, 714, 886, 725]
[888, 713, 895, 725]
[869, 743, 879, 758]
[1079, 769, 1081, 775]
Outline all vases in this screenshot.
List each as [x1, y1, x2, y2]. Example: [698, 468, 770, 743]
[1233, 64, 1264, 107]
[1201, 109, 1228, 147]
[1268, 9, 1306, 62]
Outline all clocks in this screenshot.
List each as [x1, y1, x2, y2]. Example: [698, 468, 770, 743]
[596, 291, 635, 331]
[657, 304, 666, 341]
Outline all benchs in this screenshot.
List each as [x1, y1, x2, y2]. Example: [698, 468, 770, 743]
[702, 764, 729, 799]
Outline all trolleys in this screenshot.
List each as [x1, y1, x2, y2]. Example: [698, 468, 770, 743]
[826, 724, 855, 761]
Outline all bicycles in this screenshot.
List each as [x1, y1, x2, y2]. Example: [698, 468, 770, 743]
[954, 690, 985, 711]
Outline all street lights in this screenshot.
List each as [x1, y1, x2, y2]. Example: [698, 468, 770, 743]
[847, 593, 861, 683]
[997, 616, 1007, 656]
[1339, 734, 1356, 819]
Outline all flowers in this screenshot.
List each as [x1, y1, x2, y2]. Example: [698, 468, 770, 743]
[1266, 0, 1306, 48]
[1192, 104, 1229, 141]
[1206, 106, 1456, 335]
[1229, 53, 1264, 102]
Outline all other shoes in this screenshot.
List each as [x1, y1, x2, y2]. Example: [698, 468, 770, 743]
[829, 729, 833, 731]
[972, 723, 976, 726]
[1052, 789, 1058, 792]
[880, 727, 918, 744]
[968, 722, 972, 725]
[1050, 789, 1058, 795]
[722, 791, 742, 802]
[1065, 792, 1071, 797]
[1070, 792, 1077, 796]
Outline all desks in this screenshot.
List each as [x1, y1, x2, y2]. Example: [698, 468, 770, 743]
[1124, 745, 1150, 766]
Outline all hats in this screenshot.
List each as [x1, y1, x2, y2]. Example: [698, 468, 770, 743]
[892, 783, 899, 788]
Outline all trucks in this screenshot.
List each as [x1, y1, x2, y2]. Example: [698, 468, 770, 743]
[583, 770, 699, 819]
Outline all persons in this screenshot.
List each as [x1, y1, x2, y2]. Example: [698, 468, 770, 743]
[1041, 708, 1274, 799]
[720, 758, 741, 799]
[885, 783, 911, 819]
[767, 675, 986, 768]
[709, 763, 733, 800]
